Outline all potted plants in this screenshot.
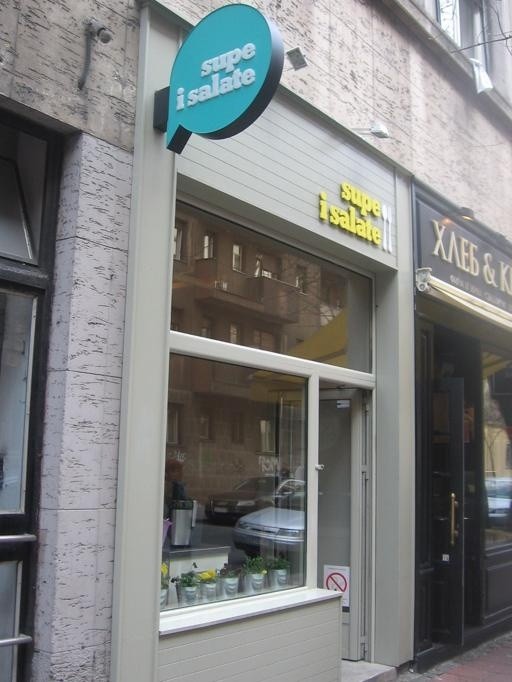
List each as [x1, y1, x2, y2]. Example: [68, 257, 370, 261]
[171, 552, 289, 608]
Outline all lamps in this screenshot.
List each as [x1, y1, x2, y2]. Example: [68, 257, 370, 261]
[350, 121, 390, 140]
[457, 206, 475, 221]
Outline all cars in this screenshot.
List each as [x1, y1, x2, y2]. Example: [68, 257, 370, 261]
[206, 475, 304, 557]
[486, 478, 512, 527]
